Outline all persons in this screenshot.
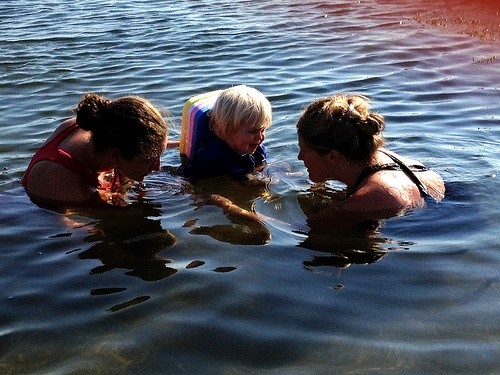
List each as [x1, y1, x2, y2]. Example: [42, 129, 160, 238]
[19, 89, 182, 210]
[172, 83, 273, 186]
[295, 92, 483, 242]
